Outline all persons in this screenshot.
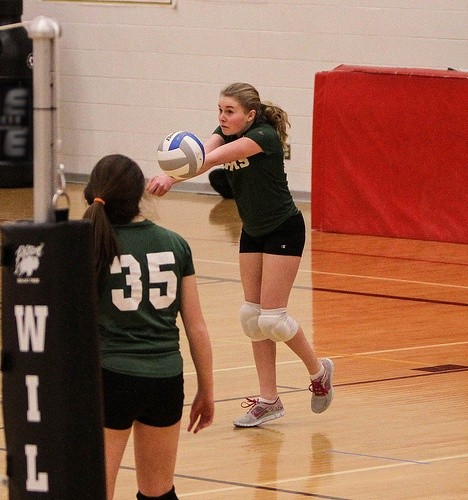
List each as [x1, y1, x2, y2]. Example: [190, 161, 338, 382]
[80, 154, 214, 500]
[146, 82, 334, 427]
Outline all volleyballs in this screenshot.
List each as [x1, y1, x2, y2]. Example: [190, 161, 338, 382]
[155, 130, 206, 180]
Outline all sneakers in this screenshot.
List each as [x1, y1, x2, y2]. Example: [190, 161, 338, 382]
[233, 396, 287, 427]
[308, 357, 334, 413]
